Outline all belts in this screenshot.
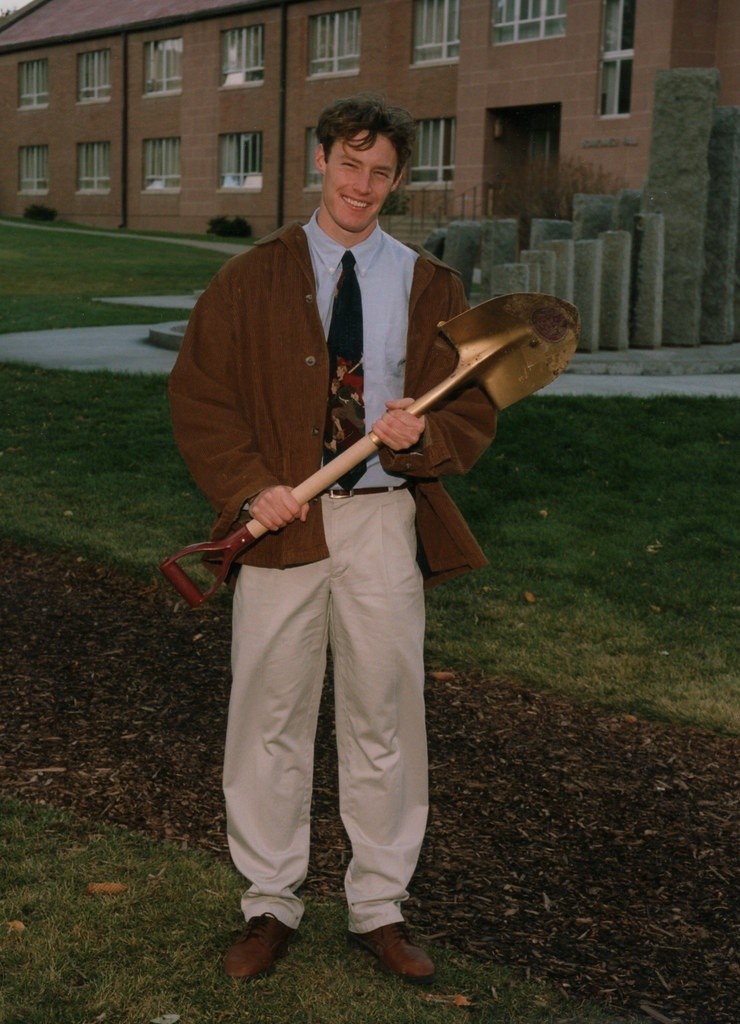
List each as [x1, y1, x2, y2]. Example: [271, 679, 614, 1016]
[320, 483, 406, 498]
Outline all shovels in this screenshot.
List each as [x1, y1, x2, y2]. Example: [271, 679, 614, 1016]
[159, 292, 580, 609]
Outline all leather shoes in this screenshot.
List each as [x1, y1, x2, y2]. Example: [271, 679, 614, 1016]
[224, 912, 299, 977]
[347, 923, 434, 984]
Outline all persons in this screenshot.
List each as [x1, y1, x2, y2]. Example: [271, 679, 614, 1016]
[166, 100, 498, 978]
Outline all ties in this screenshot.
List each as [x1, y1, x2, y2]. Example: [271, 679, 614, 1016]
[322, 250, 367, 491]
